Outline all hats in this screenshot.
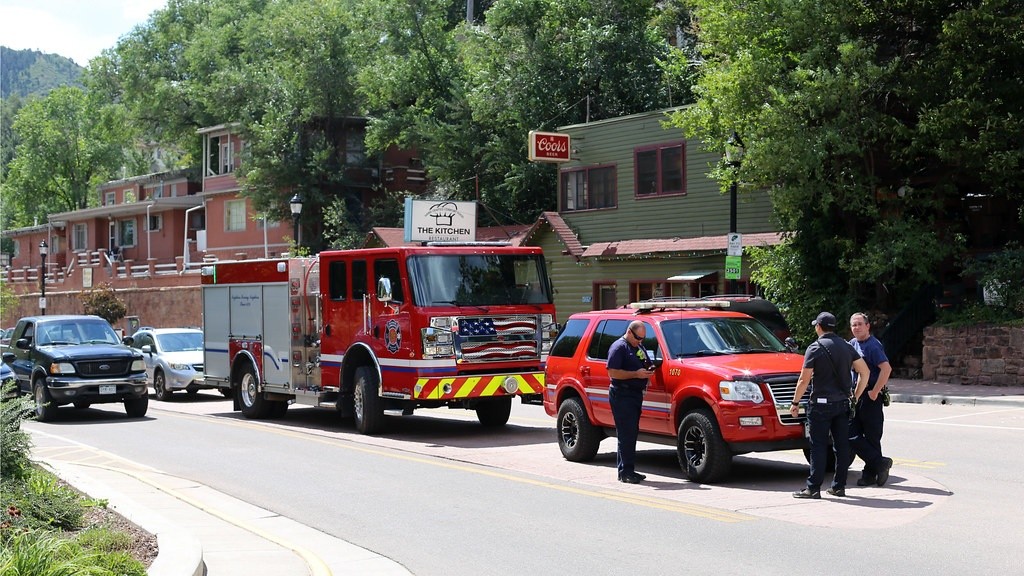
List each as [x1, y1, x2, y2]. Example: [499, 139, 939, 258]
[811, 312, 835, 327]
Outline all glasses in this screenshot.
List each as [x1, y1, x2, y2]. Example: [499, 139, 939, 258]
[630, 329, 645, 340]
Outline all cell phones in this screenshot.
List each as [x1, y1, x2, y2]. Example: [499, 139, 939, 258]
[648, 366, 657, 371]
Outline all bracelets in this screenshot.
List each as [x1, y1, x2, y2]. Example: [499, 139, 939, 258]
[791, 401, 799, 406]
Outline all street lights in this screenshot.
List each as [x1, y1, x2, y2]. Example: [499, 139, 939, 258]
[288, 191, 303, 256]
[724, 132, 746, 295]
[37, 239, 51, 317]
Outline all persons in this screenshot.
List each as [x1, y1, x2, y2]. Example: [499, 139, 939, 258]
[848, 312, 893, 487]
[606, 320, 653, 484]
[790, 312, 870, 499]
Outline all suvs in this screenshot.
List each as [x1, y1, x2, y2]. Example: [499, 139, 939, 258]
[614, 294, 796, 348]
[541, 295, 857, 483]
[2, 313, 150, 422]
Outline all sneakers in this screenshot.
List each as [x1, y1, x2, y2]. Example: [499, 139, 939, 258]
[617, 475, 640, 484]
[793, 487, 821, 499]
[633, 472, 646, 480]
[857, 479, 868, 486]
[876, 457, 893, 486]
[827, 487, 846, 497]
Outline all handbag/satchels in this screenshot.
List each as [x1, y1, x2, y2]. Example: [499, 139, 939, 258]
[883, 386, 891, 407]
[848, 397, 856, 419]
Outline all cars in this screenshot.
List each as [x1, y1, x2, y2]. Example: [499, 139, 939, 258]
[0, 327, 16, 344]
[126, 324, 234, 403]
[0, 351, 23, 403]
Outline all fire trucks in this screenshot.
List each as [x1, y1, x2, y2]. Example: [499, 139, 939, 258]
[197, 238, 560, 434]
